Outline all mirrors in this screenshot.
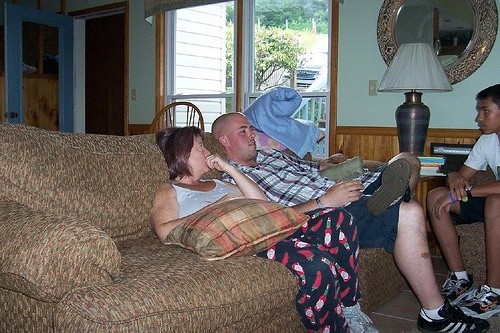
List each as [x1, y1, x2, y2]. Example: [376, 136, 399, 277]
[377, 0, 498, 87]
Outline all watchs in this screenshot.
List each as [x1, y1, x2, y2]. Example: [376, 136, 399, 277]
[464, 185, 473, 199]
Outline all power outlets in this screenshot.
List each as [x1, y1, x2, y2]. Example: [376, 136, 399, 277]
[369, 80, 378, 96]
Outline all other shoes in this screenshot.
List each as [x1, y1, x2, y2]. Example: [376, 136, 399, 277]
[342, 302, 379, 333]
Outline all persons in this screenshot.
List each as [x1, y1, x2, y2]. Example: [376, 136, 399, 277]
[151, 126, 379, 333]
[427, 84, 500, 320]
[211, 113, 490, 333]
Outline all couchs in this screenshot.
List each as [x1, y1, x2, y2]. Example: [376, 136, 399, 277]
[452, 167, 497, 287]
[0, 121, 422, 333]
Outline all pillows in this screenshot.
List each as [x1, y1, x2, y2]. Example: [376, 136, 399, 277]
[163, 198, 311, 261]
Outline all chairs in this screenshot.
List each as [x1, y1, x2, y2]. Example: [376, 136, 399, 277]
[147, 102, 205, 134]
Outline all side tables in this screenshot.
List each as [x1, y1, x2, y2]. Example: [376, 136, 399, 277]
[414, 173, 447, 249]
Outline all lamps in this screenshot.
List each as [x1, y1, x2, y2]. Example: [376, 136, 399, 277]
[377, 44, 454, 156]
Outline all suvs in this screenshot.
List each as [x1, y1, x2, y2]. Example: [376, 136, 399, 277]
[296, 52, 328, 88]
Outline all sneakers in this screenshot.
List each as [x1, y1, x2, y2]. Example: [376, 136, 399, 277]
[417, 303, 492, 333]
[367, 158, 416, 218]
[458, 284, 500, 320]
[440, 270, 475, 299]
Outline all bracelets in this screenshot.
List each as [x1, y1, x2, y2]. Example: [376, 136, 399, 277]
[315, 197, 323, 208]
[448, 191, 456, 202]
